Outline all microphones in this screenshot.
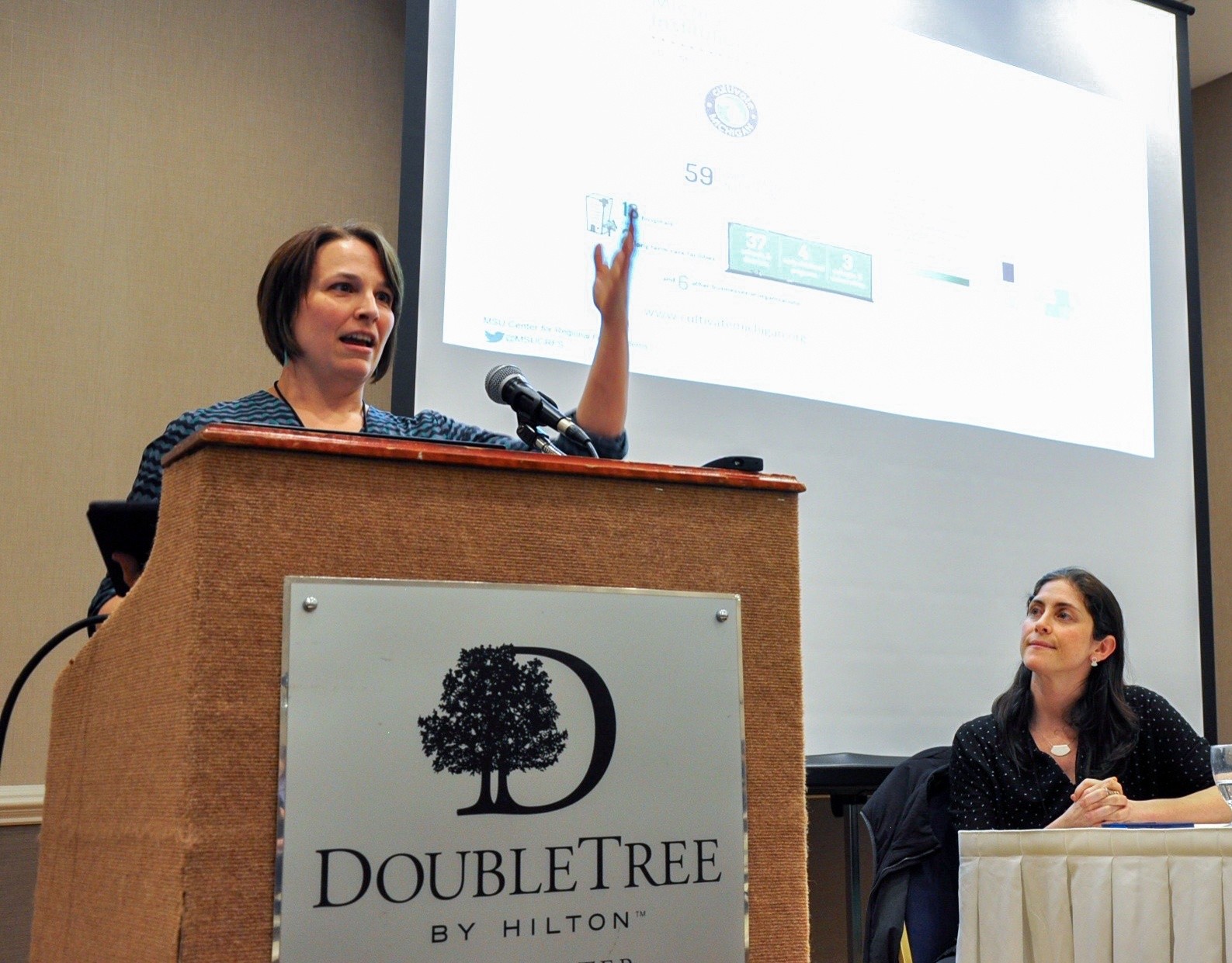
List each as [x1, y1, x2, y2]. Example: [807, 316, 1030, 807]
[485, 364, 592, 446]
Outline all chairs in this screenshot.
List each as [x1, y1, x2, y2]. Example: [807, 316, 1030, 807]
[860, 744, 960, 963]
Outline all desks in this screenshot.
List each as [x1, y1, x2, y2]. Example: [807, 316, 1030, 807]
[956, 824, 1232, 963]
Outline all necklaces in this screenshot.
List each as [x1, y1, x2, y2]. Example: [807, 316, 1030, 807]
[1028, 723, 1078, 757]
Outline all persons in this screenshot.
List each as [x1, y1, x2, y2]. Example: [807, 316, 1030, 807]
[88, 221, 637, 634]
[947, 568, 1232, 862]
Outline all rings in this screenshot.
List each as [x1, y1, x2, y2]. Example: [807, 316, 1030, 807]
[1105, 788, 1119, 796]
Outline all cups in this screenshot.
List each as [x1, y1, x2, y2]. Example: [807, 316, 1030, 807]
[1210, 744, 1232, 809]
